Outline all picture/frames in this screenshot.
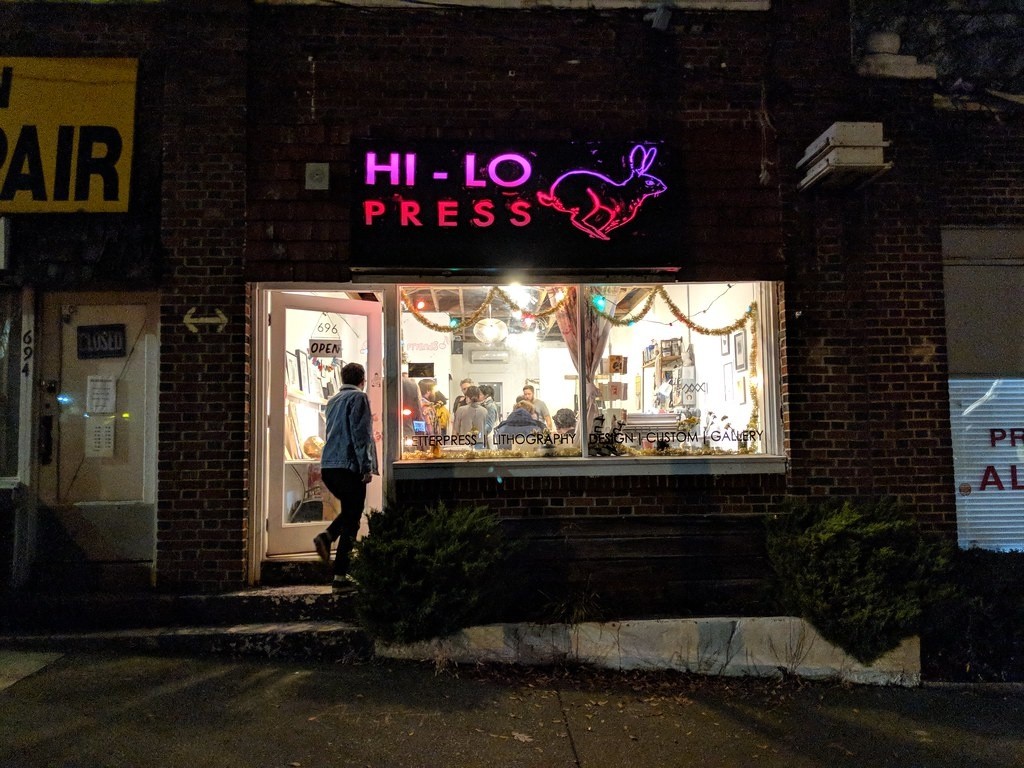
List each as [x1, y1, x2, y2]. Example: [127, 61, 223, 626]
[734, 330, 746, 370]
[295, 349, 310, 394]
[735, 376, 746, 406]
[285, 350, 301, 391]
[723, 362, 734, 402]
[720, 334, 729, 356]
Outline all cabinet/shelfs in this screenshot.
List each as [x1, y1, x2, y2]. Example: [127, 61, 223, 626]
[642, 355, 682, 413]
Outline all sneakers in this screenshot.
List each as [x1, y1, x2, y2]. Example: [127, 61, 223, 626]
[332, 578, 359, 593]
[313, 532, 331, 560]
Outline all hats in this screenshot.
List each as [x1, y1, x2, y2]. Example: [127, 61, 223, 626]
[552, 409, 576, 427]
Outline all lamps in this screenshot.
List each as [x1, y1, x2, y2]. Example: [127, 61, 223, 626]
[473, 305, 509, 343]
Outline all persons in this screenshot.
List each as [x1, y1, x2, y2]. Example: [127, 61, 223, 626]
[402, 373, 580, 451]
[314, 363, 379, 594]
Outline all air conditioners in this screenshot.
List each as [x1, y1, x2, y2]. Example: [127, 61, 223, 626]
[472, 350, 509, 364]
